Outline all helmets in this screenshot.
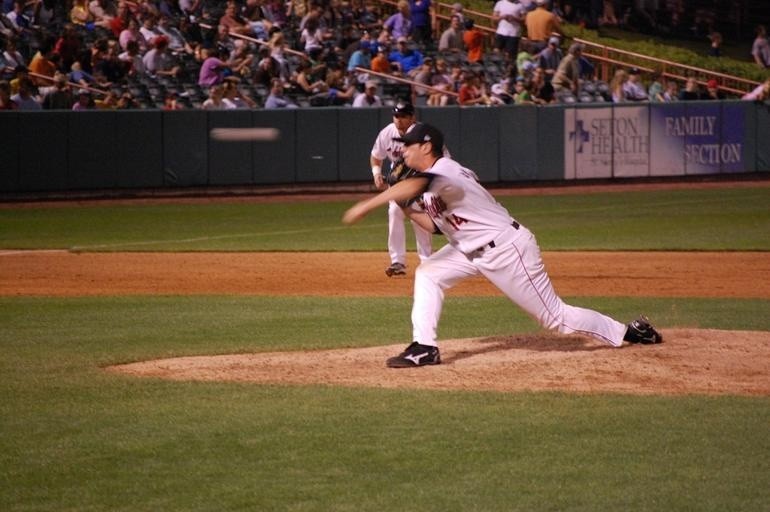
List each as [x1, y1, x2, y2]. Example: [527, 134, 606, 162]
[392, 101, 415, 115]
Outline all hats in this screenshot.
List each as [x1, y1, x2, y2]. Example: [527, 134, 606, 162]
[706, 79, 717, 88]
[366, 80, 378, 89]
[392, 122, 443, 144]
[549, 36, 560, 47]
[156, 37, 167, 47]
[397, 36, 408, 44]
[628, 68, 640, 74]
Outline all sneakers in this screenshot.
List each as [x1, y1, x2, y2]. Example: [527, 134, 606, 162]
[386, 341, 440, 367]
[630, 314, 662, 344]
[386, 263, 406, 277]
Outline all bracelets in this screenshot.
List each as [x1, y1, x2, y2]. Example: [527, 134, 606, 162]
[372, 166, 381, 176]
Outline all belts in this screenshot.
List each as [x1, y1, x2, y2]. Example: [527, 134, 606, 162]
[477, 220, 519, 254]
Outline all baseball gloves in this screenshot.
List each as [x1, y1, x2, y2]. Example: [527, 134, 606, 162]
[387, 156, 427, 210]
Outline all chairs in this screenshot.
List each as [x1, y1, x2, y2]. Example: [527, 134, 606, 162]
[1, 1, 614, 116]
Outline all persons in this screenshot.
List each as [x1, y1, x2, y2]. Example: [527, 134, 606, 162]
[742, 77, 768, 99]
[340, 123, 663, 367]
[754, 29, 770, 67]
[370, 99, 432, 275]
[2, 0, 726, 111]
